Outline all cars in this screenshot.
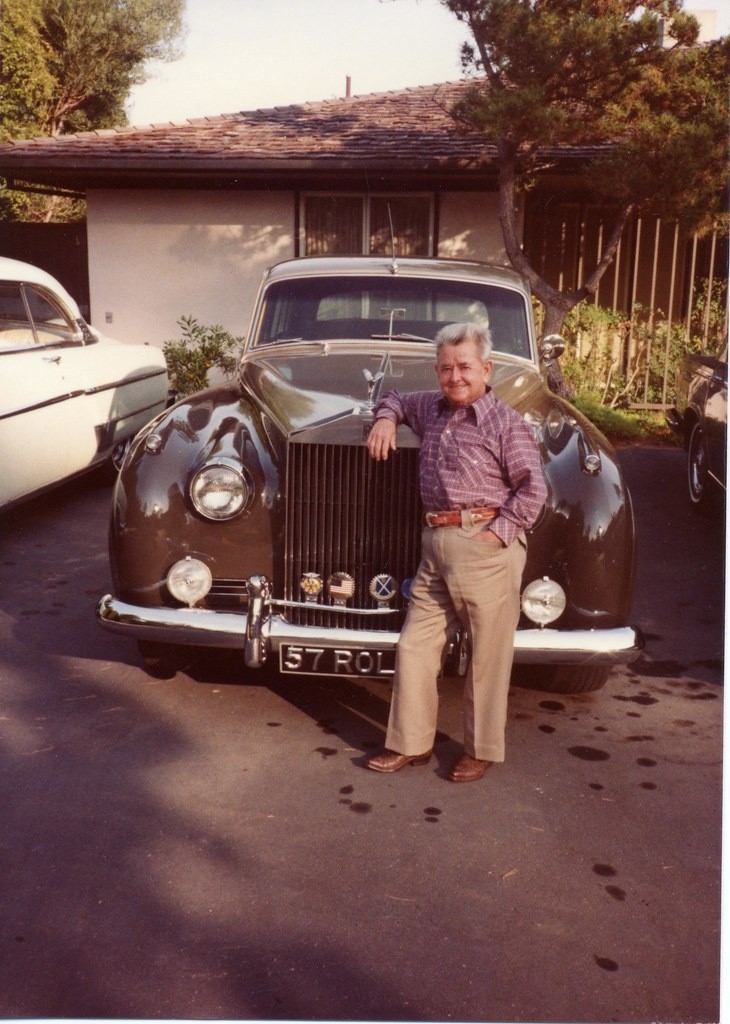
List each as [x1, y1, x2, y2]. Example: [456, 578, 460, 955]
[0, 255, 168, 528]
[99, 256, 641, 695]
[666, 334, 728, 513]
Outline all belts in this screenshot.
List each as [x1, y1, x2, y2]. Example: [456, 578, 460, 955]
[423, 507, 498, 529]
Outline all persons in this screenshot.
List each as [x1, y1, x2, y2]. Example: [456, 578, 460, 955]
[367, 322, 548, 781]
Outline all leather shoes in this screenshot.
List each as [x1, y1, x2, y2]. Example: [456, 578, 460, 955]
[448, 753, 493, 783]
[366, 749, 432, 773]
